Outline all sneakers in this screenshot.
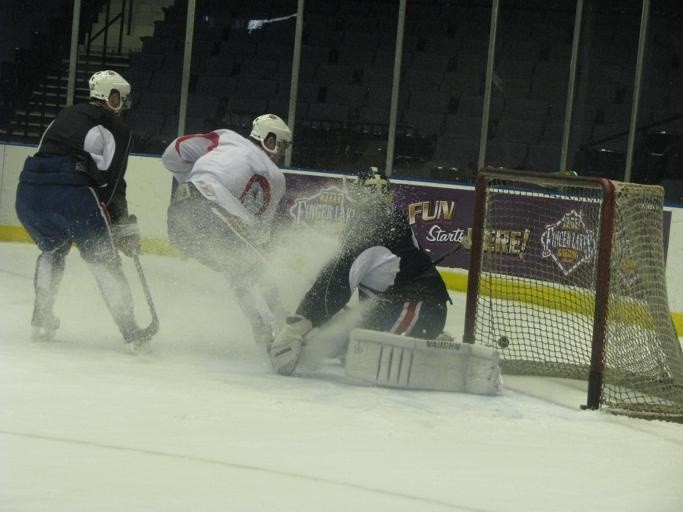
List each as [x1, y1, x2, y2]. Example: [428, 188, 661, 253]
[123, 325, 149, 344]
[30, 314, 60, 335]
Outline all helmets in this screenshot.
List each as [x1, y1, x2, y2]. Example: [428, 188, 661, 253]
[353, 166, 390, 199]
[249, 114, 292, 155]
[88, 69, 132, 111]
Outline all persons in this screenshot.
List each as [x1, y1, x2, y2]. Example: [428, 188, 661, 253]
[162, 114, 448, 376]
[15, 70, 160, 345]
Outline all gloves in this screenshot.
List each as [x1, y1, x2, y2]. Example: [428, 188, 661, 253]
[268, 313, 313, 376]
[113, 214, 143, 258]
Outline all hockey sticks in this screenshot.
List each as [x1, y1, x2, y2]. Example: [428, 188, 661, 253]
[131, 255, 159, 336]
